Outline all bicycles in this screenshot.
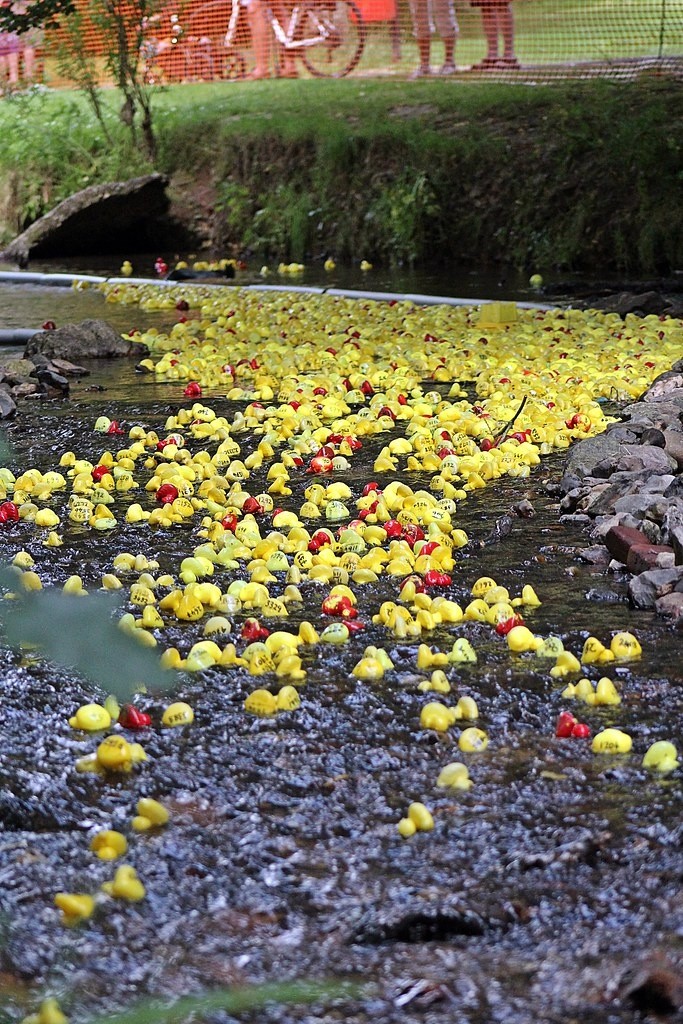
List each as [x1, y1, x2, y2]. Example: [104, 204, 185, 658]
[184, 0, 365, 81]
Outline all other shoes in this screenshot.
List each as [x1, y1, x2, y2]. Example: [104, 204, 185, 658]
[497, 58, 522, 70]
[471, 57, 496, 71]
[280, 68, 301, 79]
[437, 64, 458, 77]
[236, 67, 273, 84]
[406, 66, 437, 80]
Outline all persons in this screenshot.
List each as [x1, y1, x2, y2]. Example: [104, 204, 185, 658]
[470, 0, 521, 70]
[0, 0, 45, 96]
[409, 0, 460, 78]
[226, 0, 299, 80]
